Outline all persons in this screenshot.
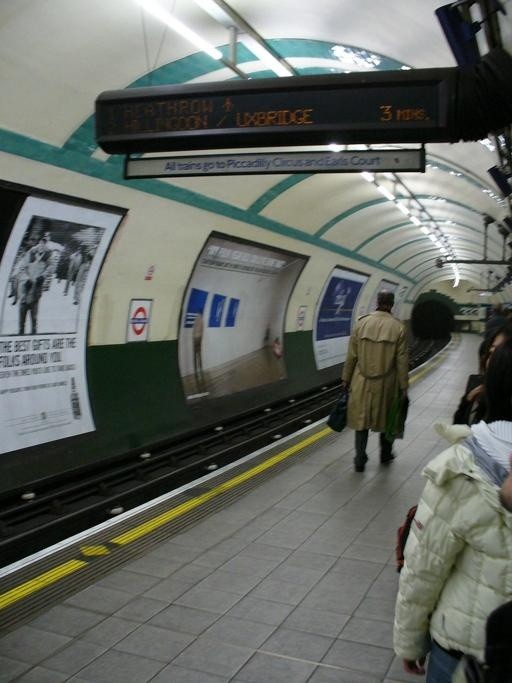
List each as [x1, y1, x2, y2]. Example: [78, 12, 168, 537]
[9, 229, 96, 336]
[192, 309, 204, 388]
[341, 289, 410, 472]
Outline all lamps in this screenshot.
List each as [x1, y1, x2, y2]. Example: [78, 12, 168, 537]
[138, 1, 293, 81]
[331, 141, 464, 290]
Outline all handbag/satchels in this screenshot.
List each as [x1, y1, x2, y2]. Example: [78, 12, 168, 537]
[327, 389, 347, 433]
